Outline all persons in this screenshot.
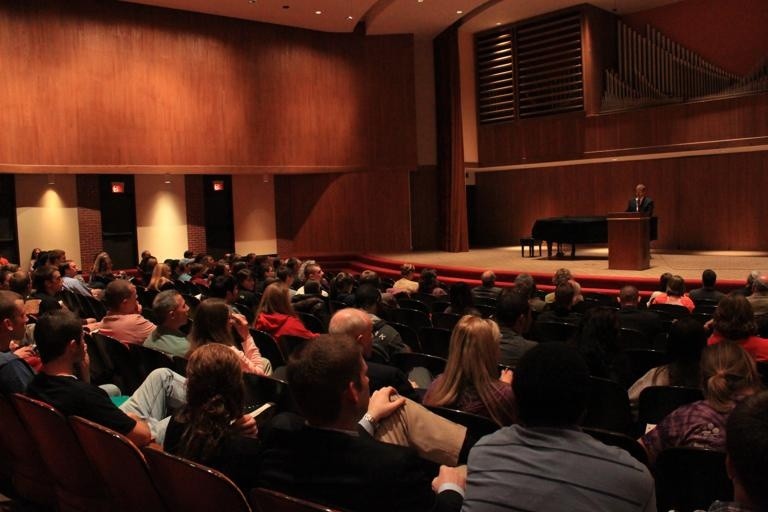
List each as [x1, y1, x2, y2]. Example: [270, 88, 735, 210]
[625, 184, 654, 212]
[0, 248, 768, 511]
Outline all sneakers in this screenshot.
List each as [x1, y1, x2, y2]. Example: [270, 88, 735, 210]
[242, 401, 277, 428]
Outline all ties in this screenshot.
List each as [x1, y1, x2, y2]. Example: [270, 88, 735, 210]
[638, 199, 640, 212]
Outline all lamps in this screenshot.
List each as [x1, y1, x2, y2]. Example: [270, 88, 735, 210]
[48, 175, 55, 185]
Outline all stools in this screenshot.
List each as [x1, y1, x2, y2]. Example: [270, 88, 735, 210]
[519, 237, 543, 258]
